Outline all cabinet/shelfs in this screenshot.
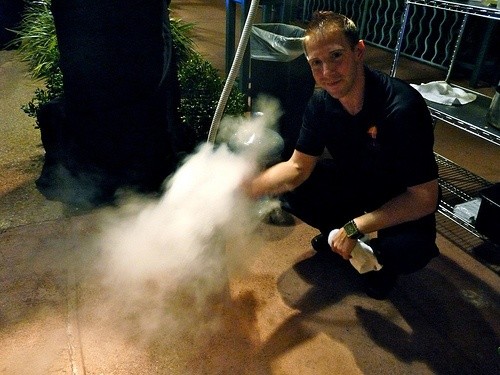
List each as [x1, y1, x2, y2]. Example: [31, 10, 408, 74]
[393, 0, 500, 251]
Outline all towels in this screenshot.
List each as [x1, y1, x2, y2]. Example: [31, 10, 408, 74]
[327, 228, 378, 274]
[409, 81, 478, 106]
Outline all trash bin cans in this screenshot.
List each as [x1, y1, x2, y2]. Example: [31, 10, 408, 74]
[48, 1, 199, 196]
[248, 22, 318, 214]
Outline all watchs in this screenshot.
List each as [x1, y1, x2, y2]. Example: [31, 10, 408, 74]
[342, 218, 365, 240]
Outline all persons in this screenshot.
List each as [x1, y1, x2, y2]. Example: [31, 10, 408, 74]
[234, 8, 443, 302]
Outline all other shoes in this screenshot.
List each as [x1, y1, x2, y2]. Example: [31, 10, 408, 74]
[362, 237, 398, 300]
[311, 232, 365, 252]
